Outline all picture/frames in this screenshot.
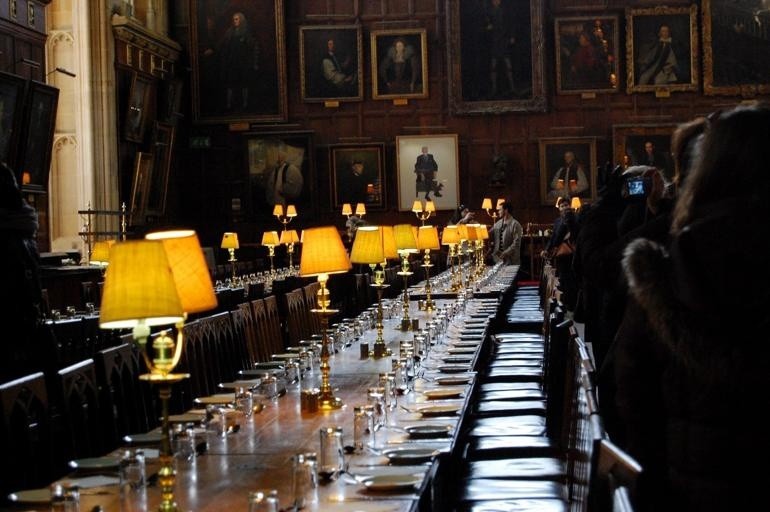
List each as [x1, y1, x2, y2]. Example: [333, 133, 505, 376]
[327, 142, 388, 211]
[538, 135, 596, 206]
[612, 125, 685, 179]
[184, 0, 288, 127]
[444, 2, 548, 116]
[370, 28, 428, 101]
[297, 23, 364, 103]
[700, 1, 768, 98]
[555, 13, 621, 96]
[239, 129, 315, 230]
[624, 4, 699, 94]
[395, 135, 459, 212]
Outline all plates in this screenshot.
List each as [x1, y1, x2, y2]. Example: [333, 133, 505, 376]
[5, 299, 501, 511]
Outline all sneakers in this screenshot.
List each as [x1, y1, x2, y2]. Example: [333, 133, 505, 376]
[425, 192, 443, 201]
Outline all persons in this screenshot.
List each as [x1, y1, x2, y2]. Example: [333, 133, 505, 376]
[639, 24, 685, 85]
[488, 199, 523, 264]
[472, 0, 517, 97]
[446, 205, 476, 269]
[413, 147, 442, 202]
[265, 152, 305, 205]
[321, 37, 353, 98]
[347, 158, 368, 205]
[204, 11, 261, 110]
[381, 39, 417, 92]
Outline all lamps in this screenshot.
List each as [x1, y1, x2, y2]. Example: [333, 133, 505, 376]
[89, 197, 522, 443]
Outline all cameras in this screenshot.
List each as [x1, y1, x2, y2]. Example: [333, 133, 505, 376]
[622, 175, 651, 199]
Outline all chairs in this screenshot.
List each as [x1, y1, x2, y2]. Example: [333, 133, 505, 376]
[438, 245, 640, 512]
[1, 246, 109, 510]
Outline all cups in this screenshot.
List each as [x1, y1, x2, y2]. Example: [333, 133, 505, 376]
[212, 265, 300, 293]
[403, 260, 521, 292]
